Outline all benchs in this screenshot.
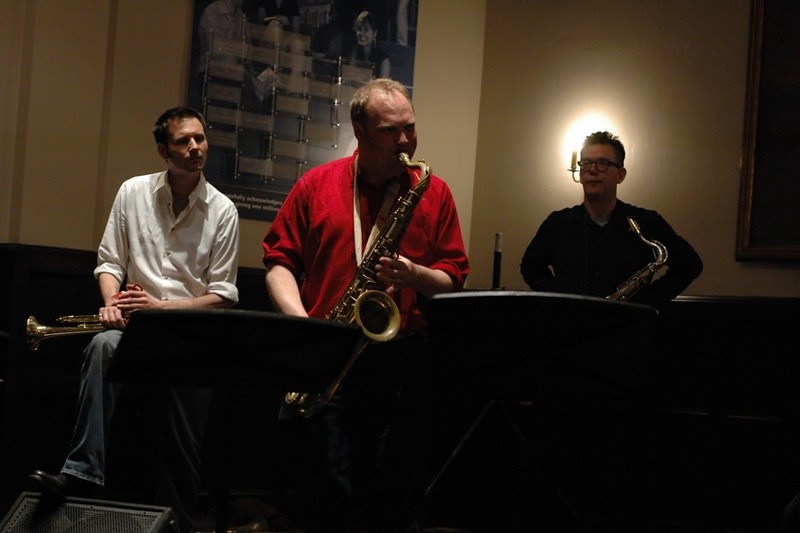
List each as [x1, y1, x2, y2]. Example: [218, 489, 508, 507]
[0, 242, 274, 491]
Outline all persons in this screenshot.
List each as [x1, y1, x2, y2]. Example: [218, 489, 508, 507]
[520, 131, 703, 309]
[338, 13, 391, 86]
[29, 106, 241, 496]
[262, 78, 471, 343]
[195, 0, 308, 104]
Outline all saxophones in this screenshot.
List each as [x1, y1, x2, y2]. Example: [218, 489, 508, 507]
[602, 215, 669, 302]
[278, 151, 431, 416]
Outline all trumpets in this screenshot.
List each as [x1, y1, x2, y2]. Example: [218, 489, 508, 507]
[26, 312, 139, 350]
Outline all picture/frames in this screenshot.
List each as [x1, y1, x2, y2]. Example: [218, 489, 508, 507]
[732, 0, 800, 265]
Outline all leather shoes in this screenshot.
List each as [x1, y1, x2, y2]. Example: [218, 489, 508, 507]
[28, 469, 82, 497]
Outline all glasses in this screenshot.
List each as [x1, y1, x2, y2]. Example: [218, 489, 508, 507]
[578, 159, 619, 172]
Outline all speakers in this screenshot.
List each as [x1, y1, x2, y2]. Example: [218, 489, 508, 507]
[0, 492, 180, 533]
